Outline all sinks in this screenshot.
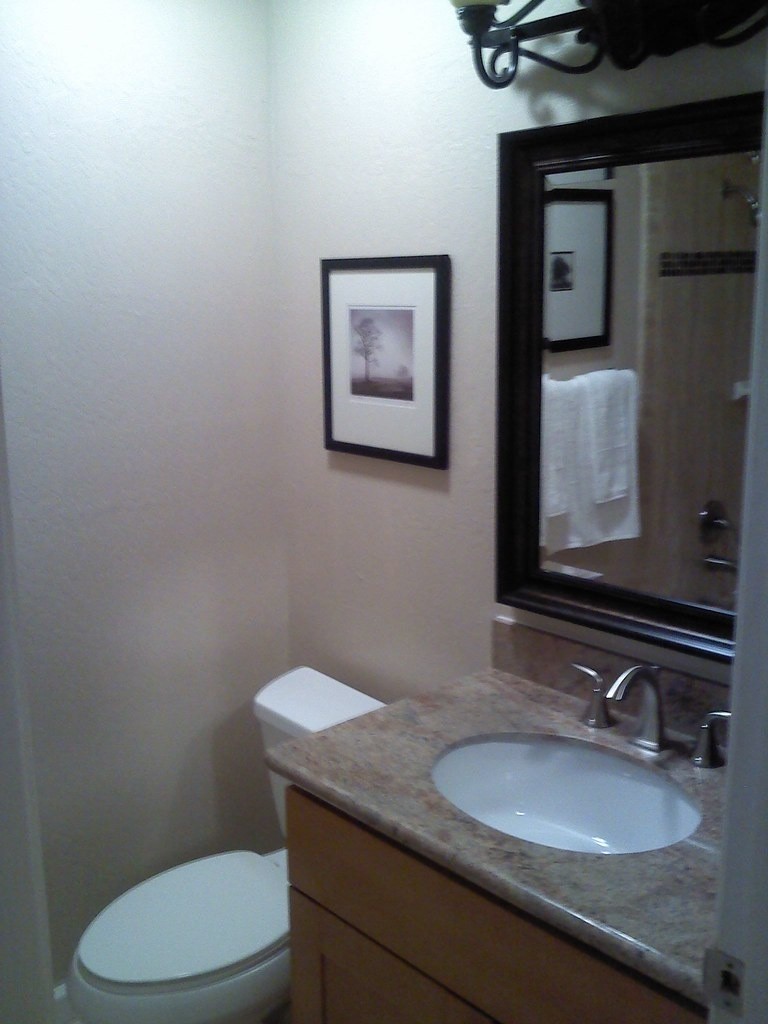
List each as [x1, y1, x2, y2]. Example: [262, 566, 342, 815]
[429, 730, 702, 855]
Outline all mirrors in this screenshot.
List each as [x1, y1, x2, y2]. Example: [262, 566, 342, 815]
[493, 90, 766, 688]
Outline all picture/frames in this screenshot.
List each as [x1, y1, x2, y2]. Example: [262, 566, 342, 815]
[319, 253, 453, 471]
[546, 189, 614, 352]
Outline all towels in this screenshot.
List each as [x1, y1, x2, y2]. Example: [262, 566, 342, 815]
[540, 370, 639, 552]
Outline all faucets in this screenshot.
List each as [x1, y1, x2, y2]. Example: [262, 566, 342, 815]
[605, 665, 662, 752]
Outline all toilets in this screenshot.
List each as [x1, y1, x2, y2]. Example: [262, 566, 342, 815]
[71, 664, 393, 1023]
